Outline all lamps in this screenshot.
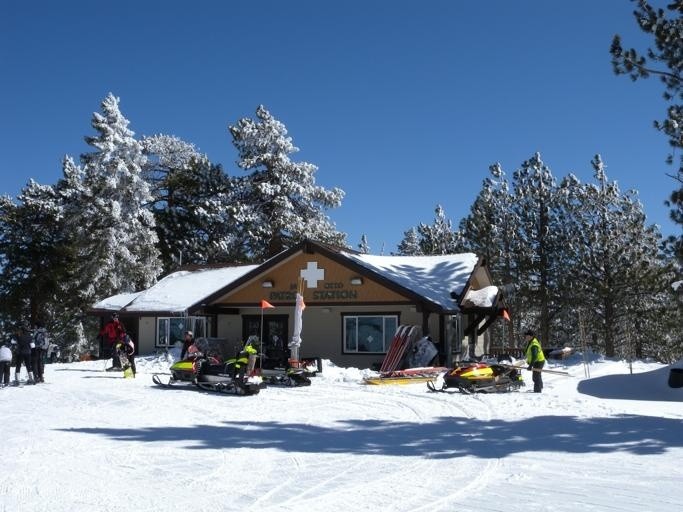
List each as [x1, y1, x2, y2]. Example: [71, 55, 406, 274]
[261, 279, 274, 289]
[350, 276, 364, 286]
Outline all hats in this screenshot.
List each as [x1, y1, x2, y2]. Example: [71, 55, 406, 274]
[523, 329, 535, 336]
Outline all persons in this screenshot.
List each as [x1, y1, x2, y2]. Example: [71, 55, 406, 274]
[0, 322, 61, 387]
[181, 330, 195, 360]
[231, 344, 249, 385]
[96, 313, 136, 379]
[524, 330, 545, 393]
